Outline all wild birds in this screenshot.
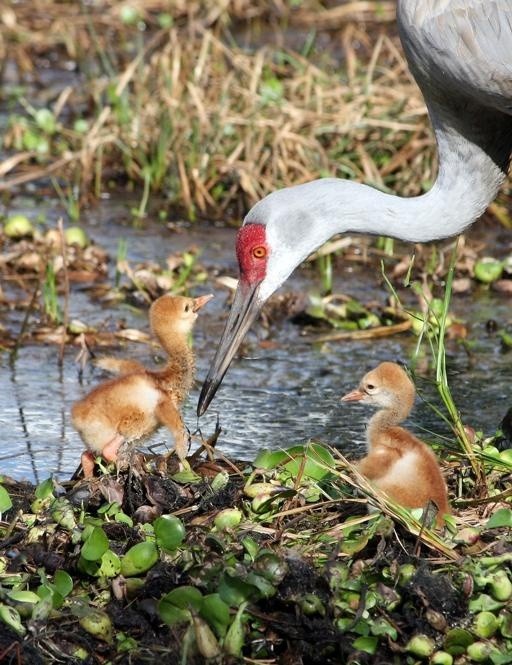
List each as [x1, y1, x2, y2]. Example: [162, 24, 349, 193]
[197, 1, 512, 417]
[68, 293, 217, 480]
[340, 361, 449, 527]
